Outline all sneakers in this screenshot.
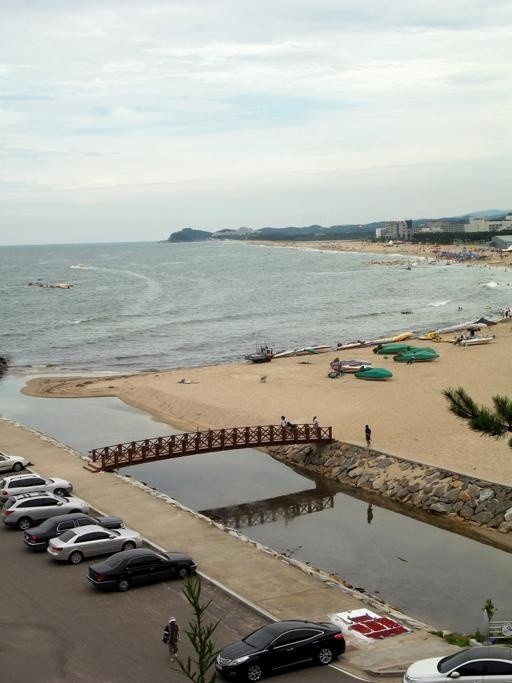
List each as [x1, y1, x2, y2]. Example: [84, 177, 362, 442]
[170, 653, 178, 661]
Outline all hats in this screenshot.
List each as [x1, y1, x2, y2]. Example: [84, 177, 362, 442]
[168, 617, 177, 624]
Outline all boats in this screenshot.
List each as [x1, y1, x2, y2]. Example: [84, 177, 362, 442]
[374, 343, 416, 353]
[458, 336, 492, 346]
[354, 367, 392, 379]
[330, 358, 371, 373]
[393, 345, 440, 362]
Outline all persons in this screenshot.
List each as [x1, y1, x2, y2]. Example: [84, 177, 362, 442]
[366, 502, 374, 524]
[280, 416, 298, 428]
[160, 616, 180, 663]
[312, 415, 320, 431]
[364, 423, 373, 447]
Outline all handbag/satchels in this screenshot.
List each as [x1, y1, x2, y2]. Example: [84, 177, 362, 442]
[163, 634, 170, 644]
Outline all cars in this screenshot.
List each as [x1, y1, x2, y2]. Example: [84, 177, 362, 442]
[0, 450, 30, 473]
[214, 618, 347, 682]
[24, 515, 124, 549]
[1, 473, 72, 502]
[1, 493, 92, 531]
[86, 547, 197, 593]
[46, 525, 142, 565]
[400, 646, 511, 682]
